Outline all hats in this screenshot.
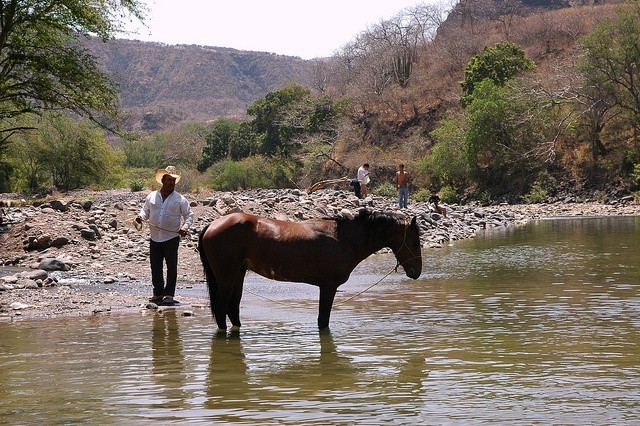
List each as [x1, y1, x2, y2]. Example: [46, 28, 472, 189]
[155, 165, 181, 185]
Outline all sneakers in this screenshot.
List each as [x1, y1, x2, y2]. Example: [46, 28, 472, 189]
[163, 295, 173, 302]
[149, 296, 163, 302]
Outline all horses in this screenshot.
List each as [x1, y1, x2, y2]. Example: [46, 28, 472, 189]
[197, 206, 422, 334]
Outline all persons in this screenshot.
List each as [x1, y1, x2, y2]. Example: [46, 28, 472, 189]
[428, 189, 446, 217]
[358, 163, 371, 198]
[136, 165, 193, 303]
[395, 164, 410, 209]
